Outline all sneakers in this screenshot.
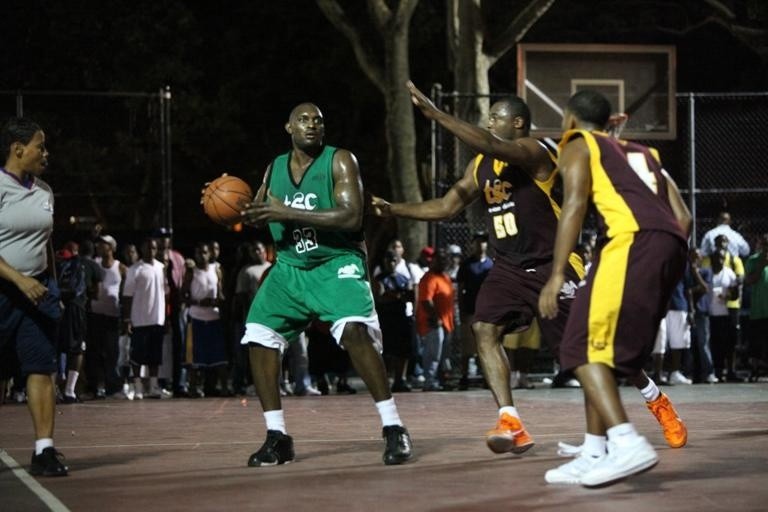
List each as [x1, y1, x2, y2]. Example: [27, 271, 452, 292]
[277, 376, 470, 396]
[544, 441, 608, 484]
[10, 378, 205, 405]
[29, 447, 69, 476]
[382, 424, 414, 465]
[645, 391, 688, 448]
[579, 435, 659, 487]
[247, 428, 295, 468]
[486, 412, 535, 454]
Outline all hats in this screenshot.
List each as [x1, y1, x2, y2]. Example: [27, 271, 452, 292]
[97, 234, 118, 249]
[446, 244, 464, 257]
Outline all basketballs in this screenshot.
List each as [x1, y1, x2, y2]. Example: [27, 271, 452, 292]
[204, 176, 253, 226]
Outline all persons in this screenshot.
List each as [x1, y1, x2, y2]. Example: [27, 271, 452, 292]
[539, 88, 694, 490]
[371, 229, 634, 392]
[3, 372, 28, 405]
[200, 102, 414, 467]
[54, 212, 359, 405]
[1, 120, 69, 478]
[373, 80, 688, 456]
[649, 211, 768, 385]
